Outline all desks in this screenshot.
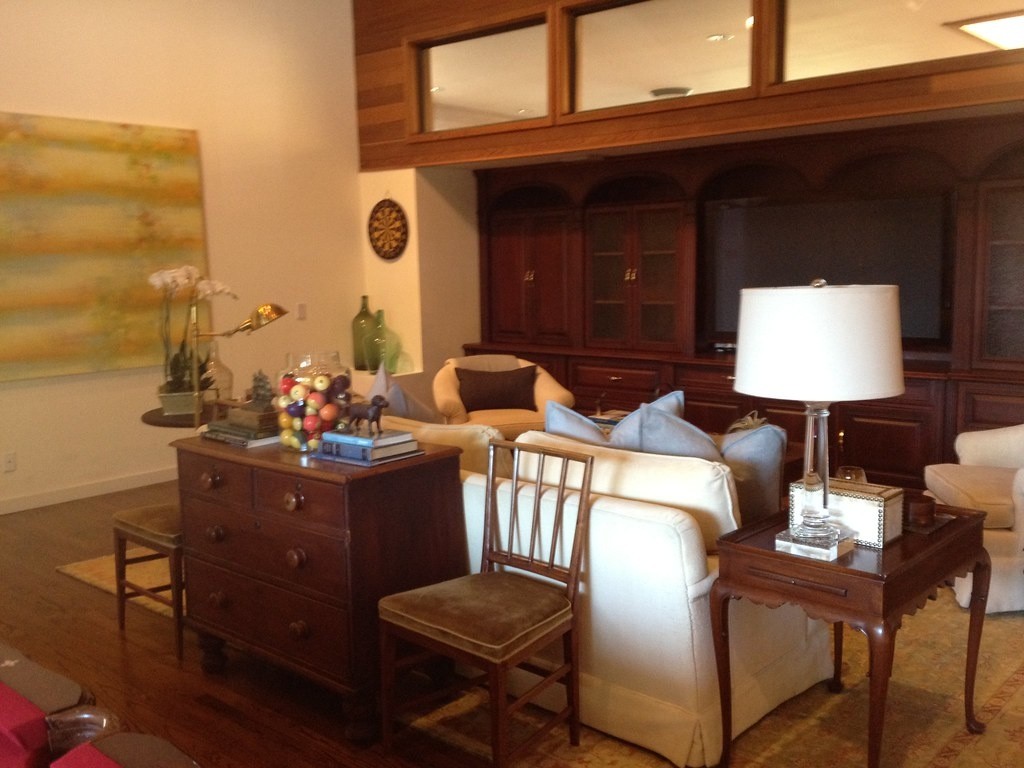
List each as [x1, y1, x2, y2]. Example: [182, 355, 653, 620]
[142, 402, 228, 428]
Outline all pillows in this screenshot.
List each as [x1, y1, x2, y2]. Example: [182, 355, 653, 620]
[454, 365, 538, 414]
[546, 389, 684, 450]
[638, 402, 788, 524]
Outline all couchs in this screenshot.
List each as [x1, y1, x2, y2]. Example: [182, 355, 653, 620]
[295, 361, 835, 768]
[923, 422, 1024, 615]
[432, 354, 575, 441]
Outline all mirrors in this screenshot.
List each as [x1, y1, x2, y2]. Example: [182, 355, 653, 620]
[421, 0, 1024, 132]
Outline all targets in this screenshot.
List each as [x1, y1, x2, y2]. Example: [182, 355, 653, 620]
[368, 199, 409, 261]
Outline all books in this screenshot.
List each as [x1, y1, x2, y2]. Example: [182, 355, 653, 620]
[307, 424, 426, 467]
[199, 421, 281, 449]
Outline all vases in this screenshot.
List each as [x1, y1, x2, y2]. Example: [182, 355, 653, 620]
[158, 380, 205, 415]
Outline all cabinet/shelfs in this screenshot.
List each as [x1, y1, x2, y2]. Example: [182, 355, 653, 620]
[463, 180, 1024, 504]
[169, 430, 464, 742]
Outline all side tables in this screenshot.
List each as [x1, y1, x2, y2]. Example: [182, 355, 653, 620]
[710, 502, 991, 768]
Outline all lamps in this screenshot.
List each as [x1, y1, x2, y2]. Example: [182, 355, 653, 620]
[734, 283, 905, 561]
[190, 302, 289, 432]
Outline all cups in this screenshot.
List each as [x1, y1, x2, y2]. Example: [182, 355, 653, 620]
[903, 493, 936, 525]
[836, 466, 867, 484]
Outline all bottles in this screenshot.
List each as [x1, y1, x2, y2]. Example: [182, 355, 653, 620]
[363, 310, 402, 374]
[199, 341, 232, 405]
[352, 296, 381, 370]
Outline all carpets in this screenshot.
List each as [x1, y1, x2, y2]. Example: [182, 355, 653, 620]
[55, 544, 1024, 767]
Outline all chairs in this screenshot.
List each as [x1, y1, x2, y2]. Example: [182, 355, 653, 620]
[113, 503, 186, 662]
[376, 436, 596, 767]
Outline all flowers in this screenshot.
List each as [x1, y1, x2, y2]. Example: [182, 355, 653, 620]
[149, 265, 238, 390]
